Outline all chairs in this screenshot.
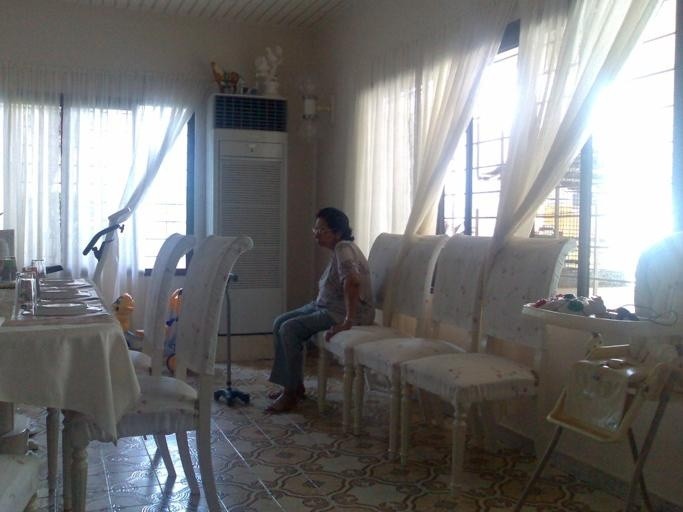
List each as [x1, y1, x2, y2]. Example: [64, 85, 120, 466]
[400, 237, 576, 469]
[128, 233, 197, 477]
[352, 233, 497, 459]
[311, 233, 412, 350]
[62, 235, 254, 512]
[318, 234, 450, 432]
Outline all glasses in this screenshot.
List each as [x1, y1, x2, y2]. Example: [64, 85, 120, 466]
[311, 226, 335, 234]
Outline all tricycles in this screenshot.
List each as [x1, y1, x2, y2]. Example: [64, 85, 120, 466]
[111, 287, 183, 373]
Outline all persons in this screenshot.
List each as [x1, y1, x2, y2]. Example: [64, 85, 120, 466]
[263, 207, 375, 414]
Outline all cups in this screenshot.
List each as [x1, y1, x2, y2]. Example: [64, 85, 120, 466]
[0, 257, 47, 321]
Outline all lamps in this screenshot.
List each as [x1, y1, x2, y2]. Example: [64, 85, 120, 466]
[303, 92, 334, 123]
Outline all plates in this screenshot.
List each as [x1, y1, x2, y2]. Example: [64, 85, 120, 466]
[38, 276, 101, 317]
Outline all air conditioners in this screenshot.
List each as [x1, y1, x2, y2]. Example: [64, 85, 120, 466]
[206, 92, 289, 335]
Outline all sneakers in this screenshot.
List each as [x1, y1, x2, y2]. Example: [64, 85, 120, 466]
[264, 389, 304, 414]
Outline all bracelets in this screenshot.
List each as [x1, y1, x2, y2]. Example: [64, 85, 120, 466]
[344, 316, 352, 323]
